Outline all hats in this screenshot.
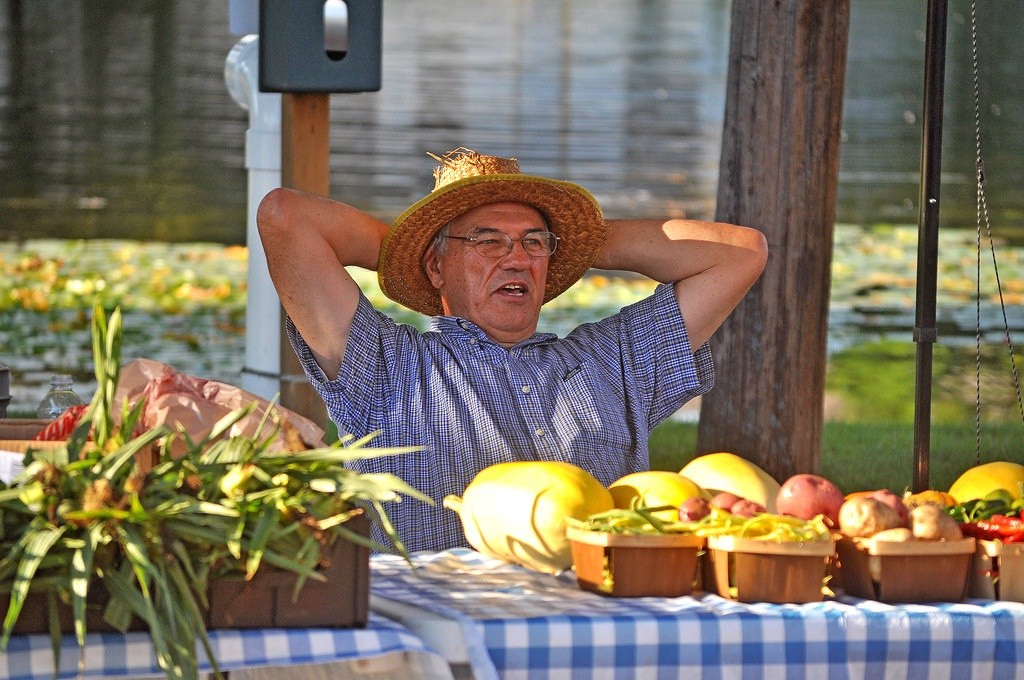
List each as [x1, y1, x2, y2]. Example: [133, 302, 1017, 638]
[377, 145, 609, 317]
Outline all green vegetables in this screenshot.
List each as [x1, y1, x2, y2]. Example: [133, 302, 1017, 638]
[557, 492, 837, 598]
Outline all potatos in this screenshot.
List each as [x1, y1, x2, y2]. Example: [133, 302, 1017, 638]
[839, 495, 964, 588]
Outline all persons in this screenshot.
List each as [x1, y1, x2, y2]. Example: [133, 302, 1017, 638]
[255, 155, 770, 556]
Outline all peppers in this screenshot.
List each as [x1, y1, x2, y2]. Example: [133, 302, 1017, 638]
[942, 487, 1024, 548]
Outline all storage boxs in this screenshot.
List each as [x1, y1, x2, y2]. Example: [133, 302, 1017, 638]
[0, 506, 371, 639]
[834, 530, 975, 605]
[566, 526, 706, 598]
[973, 539, 1024, 603]
[704, 536, 836, 605]
[0, 418, 155, 492]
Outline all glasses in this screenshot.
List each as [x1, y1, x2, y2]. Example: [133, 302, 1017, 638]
[434, 231, 560, 258]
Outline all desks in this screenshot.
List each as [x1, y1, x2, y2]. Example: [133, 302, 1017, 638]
[0, 612, 459, 680]
[370, 549, 1024, 680]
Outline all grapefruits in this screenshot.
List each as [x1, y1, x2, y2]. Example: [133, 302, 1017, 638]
[944, 459, 1024, 505]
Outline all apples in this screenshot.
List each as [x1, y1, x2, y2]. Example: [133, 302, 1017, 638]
[678, 472, 842, 528]
